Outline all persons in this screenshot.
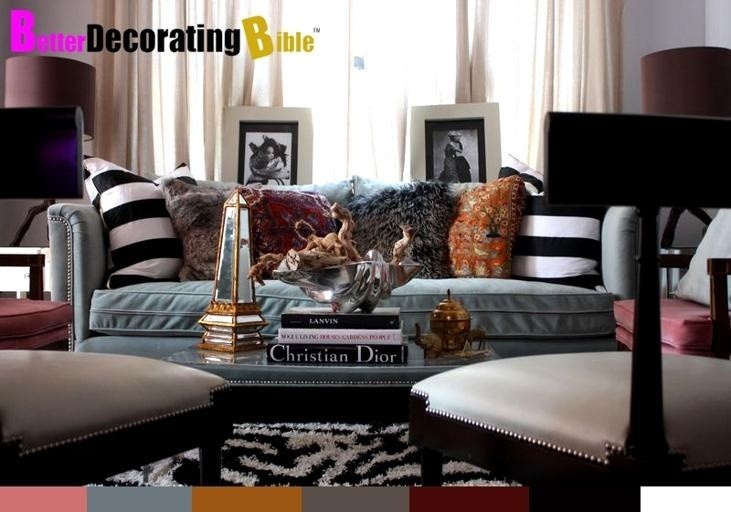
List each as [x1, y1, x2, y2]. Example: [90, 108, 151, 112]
[249, 138, 289, 184]
[444, 130, 464, 182]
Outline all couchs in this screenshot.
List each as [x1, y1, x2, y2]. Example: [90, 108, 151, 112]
[46, 179, 642, 387]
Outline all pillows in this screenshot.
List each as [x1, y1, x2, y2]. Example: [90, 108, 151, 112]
[337, 175, 468, 279]
[158, 178, 264, 283]
[448, 174, 526, 279]
[83, 153, 198, 289]
[499, 152, 609, 294]
[668, 207, 730, 310]
[235, 184, 339, 280]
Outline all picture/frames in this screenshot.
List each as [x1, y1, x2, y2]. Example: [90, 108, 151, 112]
[412, 103, 502, 183]
[220, 104, 312, 186]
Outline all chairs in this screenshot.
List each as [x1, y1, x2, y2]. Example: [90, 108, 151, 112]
[613, 252, 731, 360]
[409, 111, 731, 512]
[0, 105, 235, 487]
[0, 253, 73, 350]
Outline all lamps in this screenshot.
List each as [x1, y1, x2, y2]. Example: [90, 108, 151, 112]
[5, 55, 96, 246]
[641, 46, 730, 249]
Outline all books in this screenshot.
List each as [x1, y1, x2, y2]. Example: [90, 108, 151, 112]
[278, 324, 399, 345]
[280, 305, 403, 328]
[266, 343, 409, 366]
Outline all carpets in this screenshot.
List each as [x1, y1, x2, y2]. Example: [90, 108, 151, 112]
[84, 423, 522, 488]
